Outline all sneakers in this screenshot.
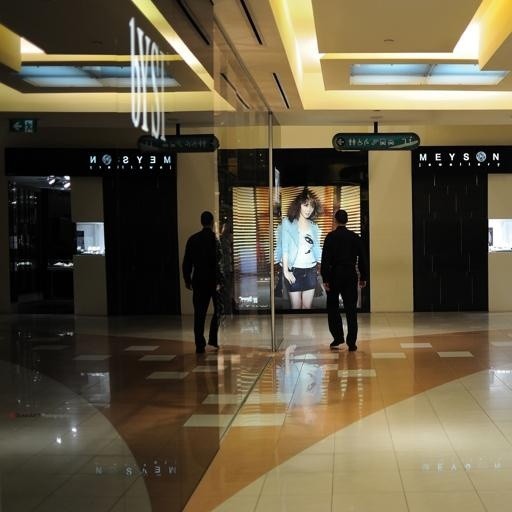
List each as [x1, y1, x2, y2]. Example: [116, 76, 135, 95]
[331, 337, 357, 351]
[193, 340, 220, 353]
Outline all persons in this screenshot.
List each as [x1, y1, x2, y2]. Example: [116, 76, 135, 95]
[182, 211, 226, 354]
[320, 210, 367, 351]
[275, 190, 321, 309]
